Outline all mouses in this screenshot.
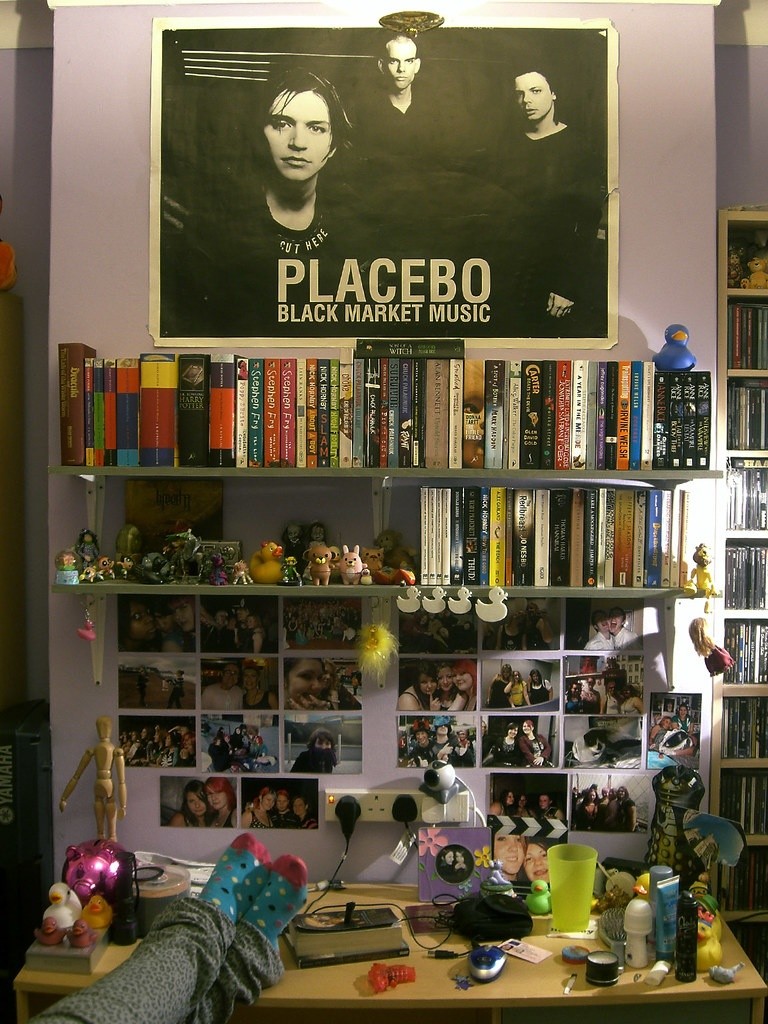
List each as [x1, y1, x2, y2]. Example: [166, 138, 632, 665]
[467, 944, 508, 983]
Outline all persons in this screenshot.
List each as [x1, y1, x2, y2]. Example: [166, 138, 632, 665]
[59, 715, 127, 842]
[472, 64, 602, 338]
[33, 832, 309, 1024]
[162, 66, 395, 338]
[374, 34, 436, 127]
[114, 595, 698, 886]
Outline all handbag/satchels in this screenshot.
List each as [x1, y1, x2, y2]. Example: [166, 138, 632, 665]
[450, 894, 533, 944]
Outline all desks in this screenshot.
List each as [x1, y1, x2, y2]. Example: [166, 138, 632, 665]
[14, 882, 768, 1024]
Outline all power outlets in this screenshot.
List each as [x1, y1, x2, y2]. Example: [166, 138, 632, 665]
[325, 787, 469, 824]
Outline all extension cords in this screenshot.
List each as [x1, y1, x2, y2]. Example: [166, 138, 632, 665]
[325, 788, 468, 824]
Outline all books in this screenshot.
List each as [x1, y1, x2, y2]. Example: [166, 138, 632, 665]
[286, 903, 410, 969]
[724, 301, 767, 908]
[58, 337, 714, 588]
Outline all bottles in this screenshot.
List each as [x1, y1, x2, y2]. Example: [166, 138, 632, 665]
[649, 866, 673, 944]
[674, 891, 698, 982]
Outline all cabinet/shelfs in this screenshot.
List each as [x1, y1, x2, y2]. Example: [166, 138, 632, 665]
[716, 206, 768, 967]
[46, 464, 724, 598]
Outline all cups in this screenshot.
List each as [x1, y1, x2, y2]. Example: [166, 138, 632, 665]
[548, 843, 598, 932]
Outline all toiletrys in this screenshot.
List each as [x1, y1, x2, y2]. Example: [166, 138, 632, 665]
[648, 865, 698, 983]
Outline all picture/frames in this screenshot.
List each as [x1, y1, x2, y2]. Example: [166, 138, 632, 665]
[418, 827, 492, 902]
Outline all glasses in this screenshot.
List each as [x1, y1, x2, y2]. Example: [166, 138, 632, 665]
[222, 669, 239, 679]
